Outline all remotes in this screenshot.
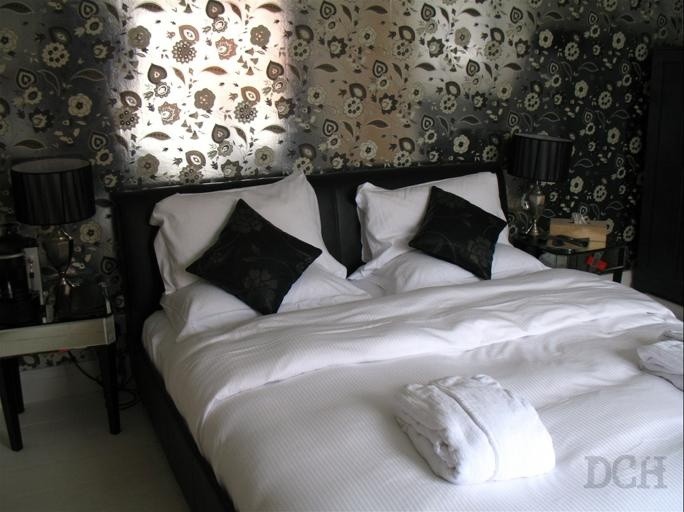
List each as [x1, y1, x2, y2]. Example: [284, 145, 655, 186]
[556, 234, 588, 248]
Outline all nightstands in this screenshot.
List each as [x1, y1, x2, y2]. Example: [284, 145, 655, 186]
[511, 234, 626, 283]
[0, 300, 123, 451]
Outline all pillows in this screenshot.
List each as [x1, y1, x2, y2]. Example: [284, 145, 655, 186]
[408, 186, 508, 282]
[350, 172, 513, 280]
[365, 243, 550, 295]
[159, 266, 373, 344]
[185, 199, 322, 316]
[150, 168, 348, 295]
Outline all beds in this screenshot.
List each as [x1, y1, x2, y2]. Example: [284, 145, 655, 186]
[107, 160, 684, 512]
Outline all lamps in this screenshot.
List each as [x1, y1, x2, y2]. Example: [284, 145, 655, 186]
[507, 132, 571, 236]
[11, 157, 96, 312]
